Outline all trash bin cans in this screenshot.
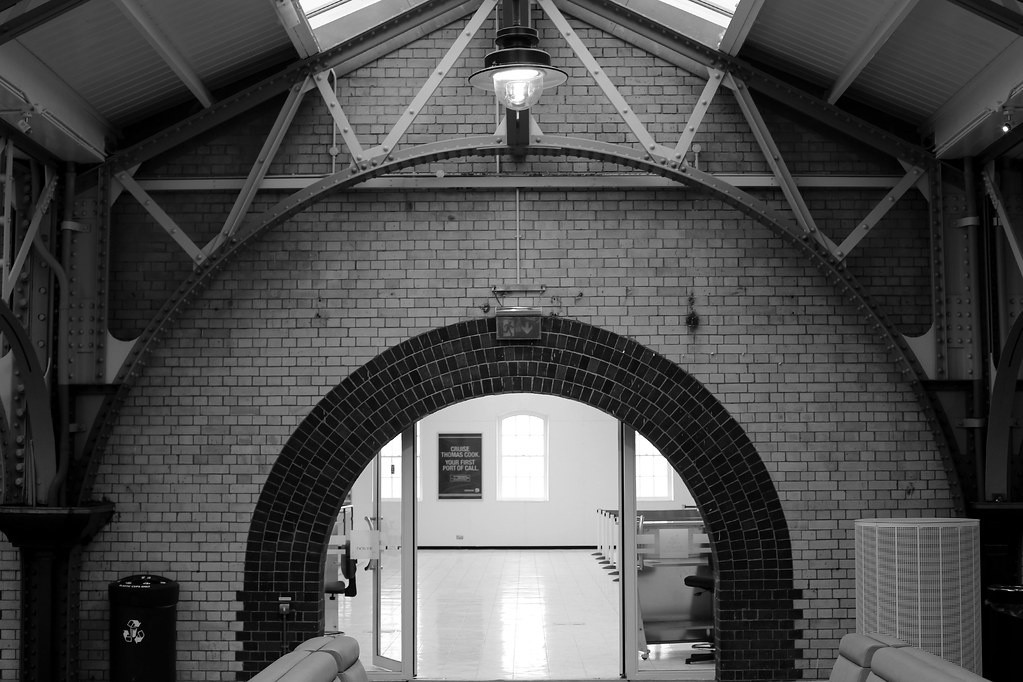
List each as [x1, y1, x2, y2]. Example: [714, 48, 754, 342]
[109, 575, 179, 682]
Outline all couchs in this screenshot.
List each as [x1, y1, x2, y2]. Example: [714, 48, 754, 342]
[247, 636, 368, 682]
[829, 632, 993, 682]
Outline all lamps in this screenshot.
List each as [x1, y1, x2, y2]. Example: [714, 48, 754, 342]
[468, 0, 569, 111]
